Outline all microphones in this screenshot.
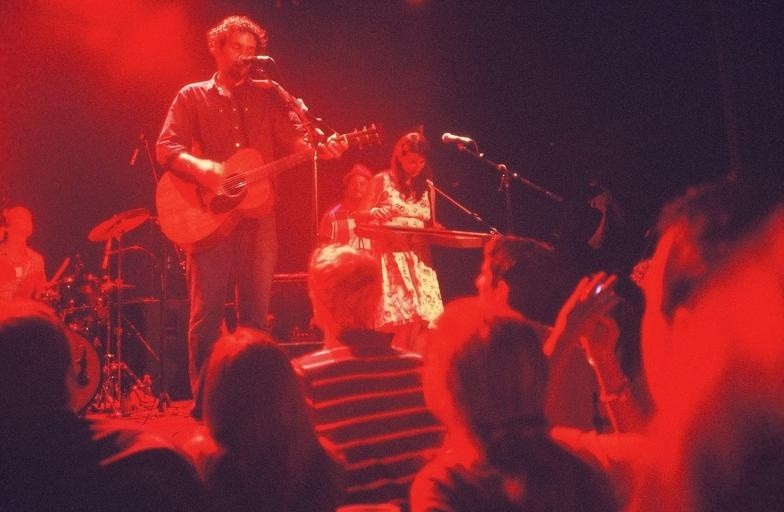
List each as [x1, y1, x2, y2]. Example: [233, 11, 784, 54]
[129, 129, 146, 165]
[235, 52, 274, 69]
[441, 129, 480, 156]
[423, 175, 438, 194]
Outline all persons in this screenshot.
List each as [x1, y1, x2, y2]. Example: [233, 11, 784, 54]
[313, 164, 373, 252]
[422, 235, 596, 459]
[1, 206, 54, 317]
[367, 131, 446, 330]
[625, 207, 783, 512]
[408, 316, 623, 512]
[538, 180, 774, 501]
[291, 242, 448, 511]
[197, 328, 348, 510]
[568, 168, 645, 305]
[0, 312, 202, 512]
[155, 16, 348, 420]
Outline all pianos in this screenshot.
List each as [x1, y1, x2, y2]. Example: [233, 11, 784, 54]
[354, 220, 552, 255]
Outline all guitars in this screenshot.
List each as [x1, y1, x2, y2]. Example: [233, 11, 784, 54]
[155, 122, 380, 255]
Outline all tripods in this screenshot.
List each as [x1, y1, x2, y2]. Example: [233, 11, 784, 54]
[89, 237, 153, 418]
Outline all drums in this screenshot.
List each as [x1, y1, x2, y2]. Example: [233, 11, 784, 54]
[45, 275, 95, 313]
[65, 328, 105, 416]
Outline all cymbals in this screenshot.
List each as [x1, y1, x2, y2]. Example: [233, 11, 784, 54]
[87, 208, 149, 242]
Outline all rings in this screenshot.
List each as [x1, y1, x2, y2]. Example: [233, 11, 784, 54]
[596, 284, 608, 296]
